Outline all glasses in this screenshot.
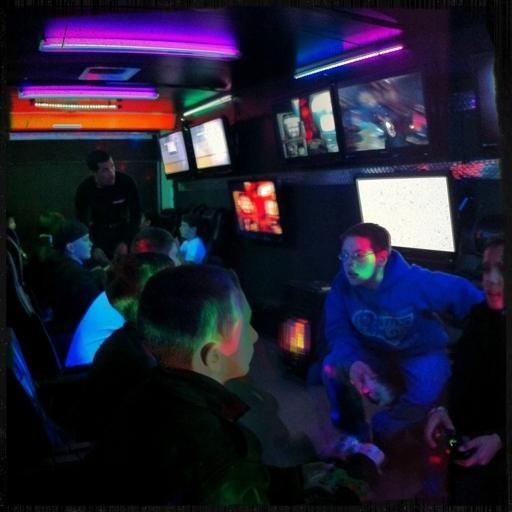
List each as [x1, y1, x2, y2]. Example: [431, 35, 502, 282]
[338, 250, 375, 260]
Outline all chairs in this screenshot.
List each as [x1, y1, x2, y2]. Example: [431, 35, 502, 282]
[3, 228, 97, 469]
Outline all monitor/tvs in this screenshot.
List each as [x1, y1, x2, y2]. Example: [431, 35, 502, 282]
[190, 114, 236, 175]
[157, 128, 190, 178]
[334, 62, 437, 160]
[352, 170, 461, 257]
[273, 83, 344, 172]
[227, 177, 289, 238]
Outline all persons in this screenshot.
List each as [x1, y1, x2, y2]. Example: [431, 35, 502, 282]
[72, 147, 140, 261]
[283, 115, 301, 139]
[5, 208, 512, 512]
